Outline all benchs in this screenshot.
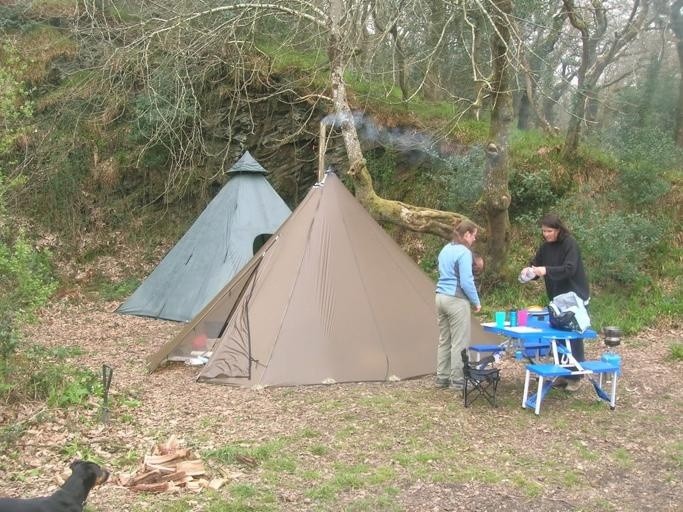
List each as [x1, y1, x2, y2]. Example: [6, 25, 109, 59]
[469, 342, 619, 415]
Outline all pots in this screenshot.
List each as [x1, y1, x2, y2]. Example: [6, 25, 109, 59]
[604, 325, 624, 338]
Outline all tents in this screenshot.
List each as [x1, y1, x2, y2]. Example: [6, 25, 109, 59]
[146, 163, 505, 392]
[114, 150, 294, 324]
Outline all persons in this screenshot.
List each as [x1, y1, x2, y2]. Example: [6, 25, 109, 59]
[519, 213, 590, 391]
[433, 220, 483, 389]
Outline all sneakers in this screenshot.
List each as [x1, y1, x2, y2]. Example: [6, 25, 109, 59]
[555, 377, 583, 392]
[433, 374, 480, 391]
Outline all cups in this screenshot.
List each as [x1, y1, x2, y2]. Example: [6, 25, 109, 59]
[496, 312, 506, 329]
[509, 310, 517, 327]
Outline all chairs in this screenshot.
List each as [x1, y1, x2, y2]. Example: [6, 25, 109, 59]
[460, 348, 501, 409]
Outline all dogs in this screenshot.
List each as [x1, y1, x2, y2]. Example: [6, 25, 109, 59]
[0, 459, 109, 512]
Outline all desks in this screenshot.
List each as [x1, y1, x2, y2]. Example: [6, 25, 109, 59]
[478, 314, 598, 410]
